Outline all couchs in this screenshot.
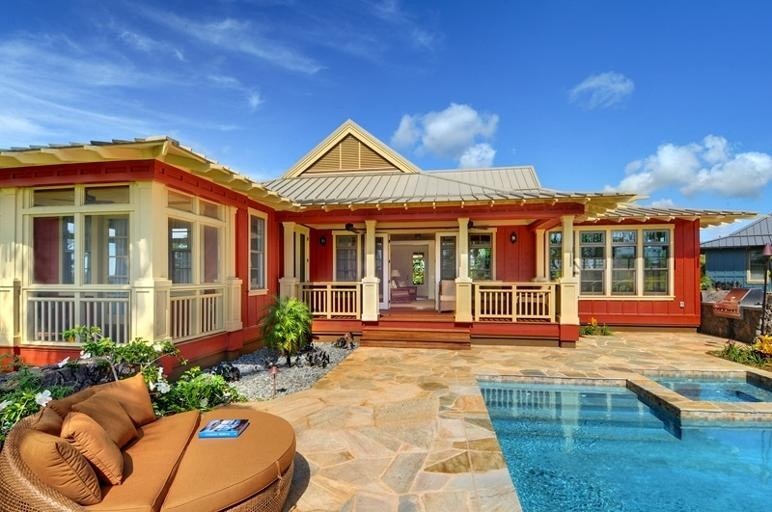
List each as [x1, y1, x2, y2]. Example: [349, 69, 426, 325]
[390, 280, 417, 303]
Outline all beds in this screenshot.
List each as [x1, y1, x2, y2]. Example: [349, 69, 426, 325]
[1, 373, 296, 511]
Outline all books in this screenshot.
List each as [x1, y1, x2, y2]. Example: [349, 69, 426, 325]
[196, 418, 250, 438]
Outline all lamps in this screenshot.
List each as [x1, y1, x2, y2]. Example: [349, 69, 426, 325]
[391, 270, 400, 282]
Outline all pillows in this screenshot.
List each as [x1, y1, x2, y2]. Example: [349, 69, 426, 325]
[19, 374, 158, 506]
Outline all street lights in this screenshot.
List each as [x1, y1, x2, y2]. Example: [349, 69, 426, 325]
[761, 243, 772, 335]
